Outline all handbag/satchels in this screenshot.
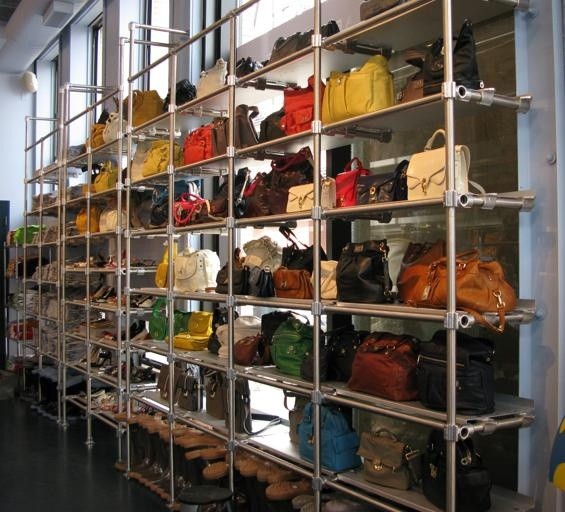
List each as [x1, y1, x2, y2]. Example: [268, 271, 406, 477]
[281, 74, 327, 136]
[154, 242, 329, 302]
[285, 389, 498, 512]
[259, 108, 287, 142]
[156, 363, 253, 434]
[335, 243, 395, 304]
[321, 54, 396, 126]
[345, 331, 417, 402]
[406, 128, 471, 201]
[399, 20, 486, 105]
[234, 147, 361, 216]
[414, 330, 497, 415]
[7, 226, 62, 356]
[213, 103, 259, 155]
[355, 159, 409, 206]
[263, 21, 340, 65]
[301, 327, 350, 385]
[74, 58, 229, 233]
[395, 242, 516, 334]
[310, 260, 338, 301]
[151, 296, 308, 378]
[235, 56, 263, 78]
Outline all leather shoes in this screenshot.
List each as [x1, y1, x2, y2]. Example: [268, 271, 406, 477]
[63, 250, 155, 382]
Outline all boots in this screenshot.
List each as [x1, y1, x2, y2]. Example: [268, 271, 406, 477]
[20, 363, 87, 421]
[110, 411, 311, 511]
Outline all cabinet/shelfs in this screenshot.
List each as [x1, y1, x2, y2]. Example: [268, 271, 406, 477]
[26, 43, 121, 466]
[6, 218, 39, 388]
[133, 1, 537, 508]
